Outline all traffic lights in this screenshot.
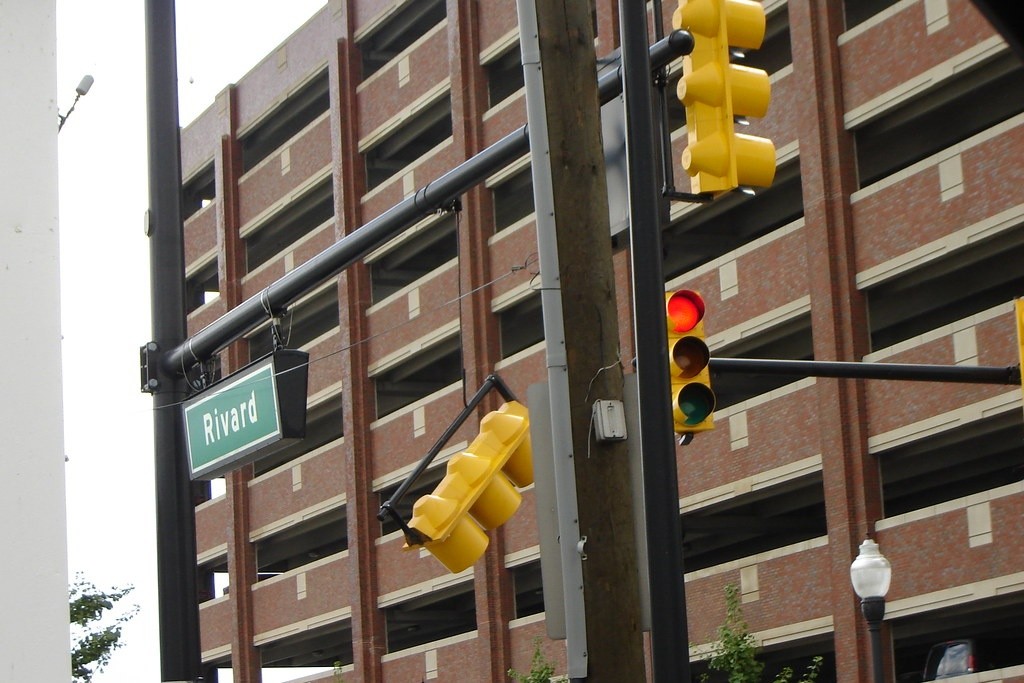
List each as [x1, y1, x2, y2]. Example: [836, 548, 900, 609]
[665, 288, 715, 435]
[401, 400, 535, 574]
[672, 1, 778, 197]
